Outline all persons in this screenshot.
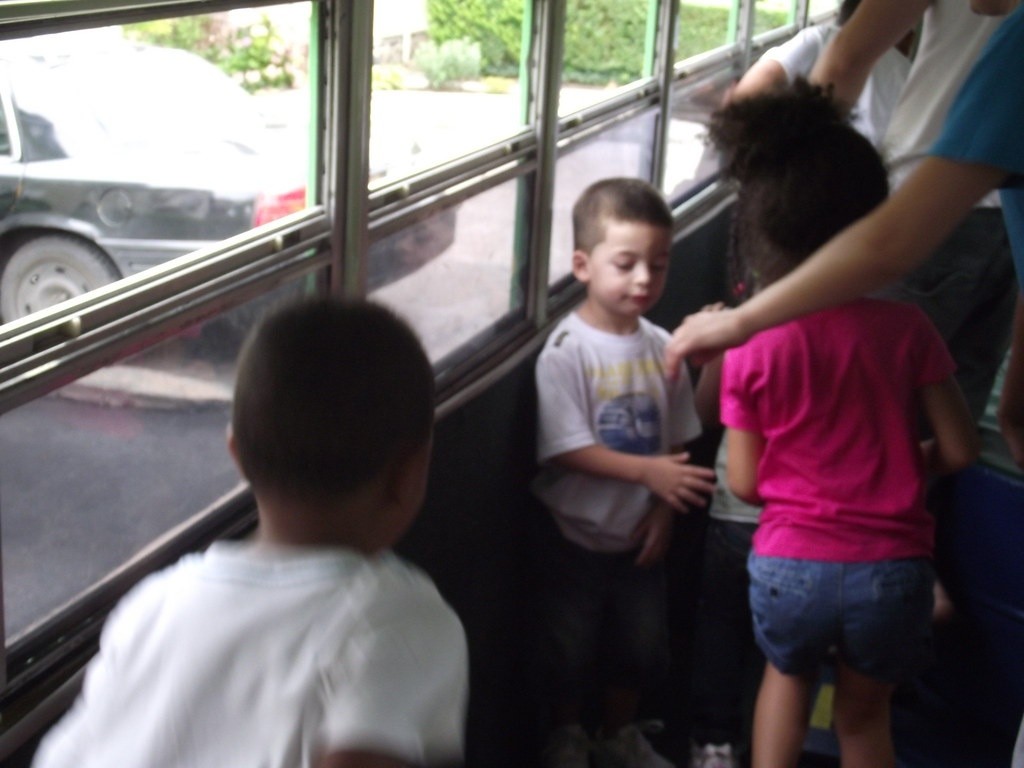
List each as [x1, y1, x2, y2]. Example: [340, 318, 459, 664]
[694, 187, 768, 768]
[705, 70, 978, 768]
[534, 178, 718, 768]
[721, 0, 916, 148]
[30, 291, 469, 768]
[808, 0, 1024, 606]
[661, 0, 1024, 766]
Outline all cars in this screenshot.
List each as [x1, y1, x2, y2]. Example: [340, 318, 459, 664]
[0, 32, 463, 322]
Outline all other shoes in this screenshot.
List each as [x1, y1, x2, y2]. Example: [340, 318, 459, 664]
[593, 723, 676, 768]
[541, 725, 590, 768]
[687, 721, 748, 768]
